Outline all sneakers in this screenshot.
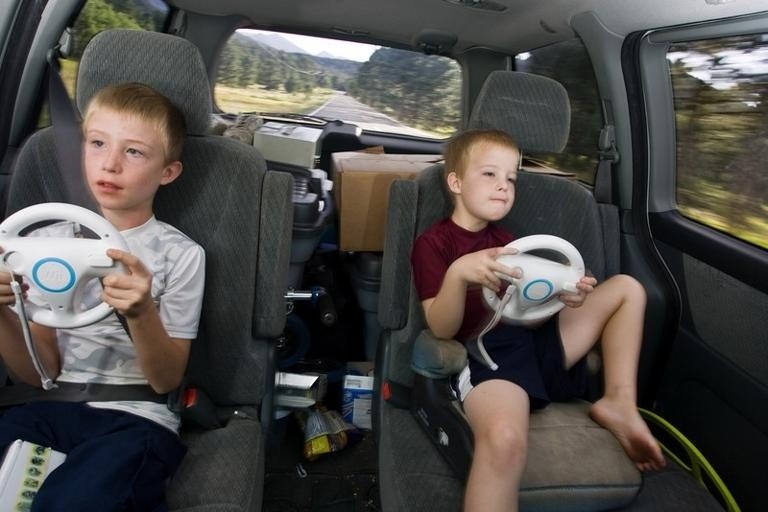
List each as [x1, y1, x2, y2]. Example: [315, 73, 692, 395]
[222, 112, 263, 146]
[204, 110, 234, 135]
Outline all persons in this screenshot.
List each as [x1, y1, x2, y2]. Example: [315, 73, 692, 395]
[0, 80, 212, 510]
[411, 127, 669, 511]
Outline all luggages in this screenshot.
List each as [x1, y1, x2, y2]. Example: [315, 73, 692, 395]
[274, 228, 383, 368]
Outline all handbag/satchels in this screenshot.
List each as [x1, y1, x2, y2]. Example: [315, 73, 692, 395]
[264, 455, 377, 512]
